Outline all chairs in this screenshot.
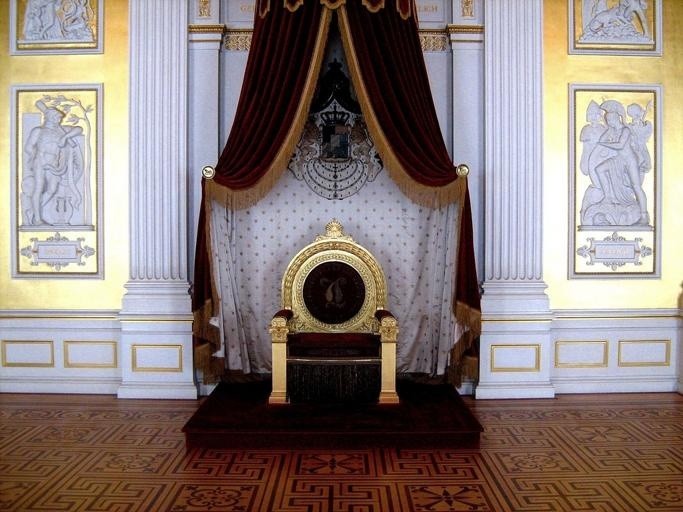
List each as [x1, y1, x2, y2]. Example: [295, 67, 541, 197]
[268, 217, 400, 406]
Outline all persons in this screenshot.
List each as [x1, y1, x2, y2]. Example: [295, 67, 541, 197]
[20, 107, 84, 227]
[623, 102, 653, 187]
[578, 109, 652, 226]
[579, 99, 606, 180]
